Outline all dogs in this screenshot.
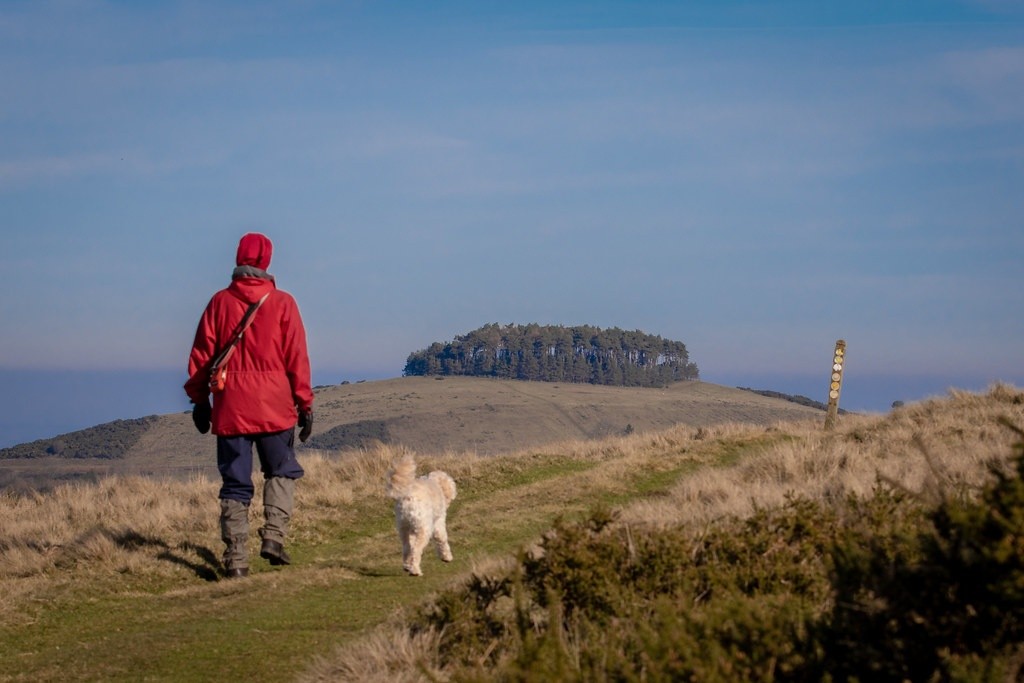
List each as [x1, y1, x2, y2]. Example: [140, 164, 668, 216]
[387, 454, 459, 577]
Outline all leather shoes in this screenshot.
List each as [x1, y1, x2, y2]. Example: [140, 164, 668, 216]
[260, 538, 289, 565]
[227, 568, 248, 578]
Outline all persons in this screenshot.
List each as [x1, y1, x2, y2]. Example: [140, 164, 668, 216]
[183, 232, 314, 580]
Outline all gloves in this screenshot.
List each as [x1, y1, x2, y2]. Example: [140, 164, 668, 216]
[193, 402, 211, 434]
[298, 408, 313, 442]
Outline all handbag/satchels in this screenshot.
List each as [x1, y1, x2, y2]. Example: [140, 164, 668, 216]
[208, 366, 224, 392]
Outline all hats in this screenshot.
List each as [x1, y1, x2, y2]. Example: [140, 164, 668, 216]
[235, 234, 273, 269]
